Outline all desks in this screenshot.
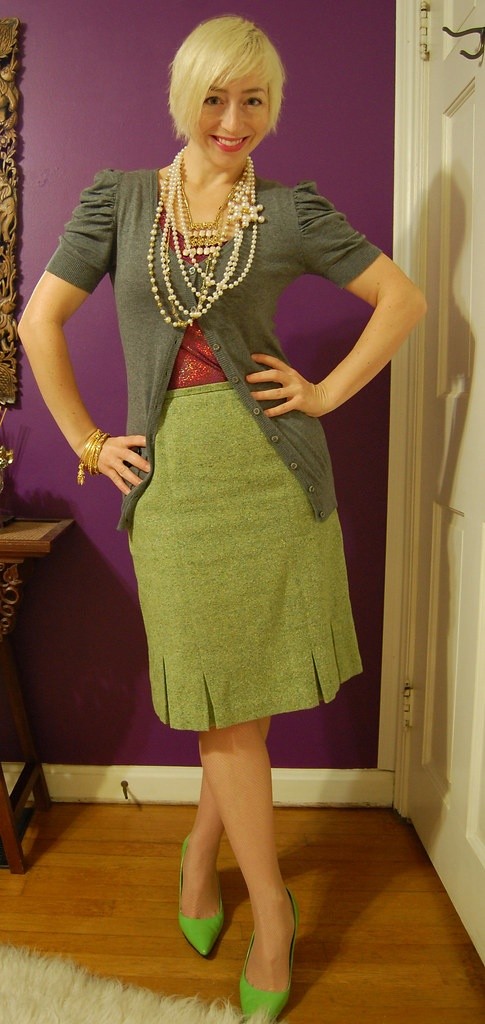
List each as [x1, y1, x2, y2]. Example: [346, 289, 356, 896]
[0, 517, 76, 875]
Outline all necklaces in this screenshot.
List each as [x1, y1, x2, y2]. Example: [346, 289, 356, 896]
[147, 146, 265, 327]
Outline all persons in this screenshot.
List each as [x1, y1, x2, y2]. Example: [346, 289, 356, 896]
[16, 17, 428, 1024]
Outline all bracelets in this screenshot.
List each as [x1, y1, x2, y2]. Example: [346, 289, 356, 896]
[77, 428, 112, 486]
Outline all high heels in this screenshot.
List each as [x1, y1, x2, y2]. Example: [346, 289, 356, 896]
[176, 832, 225, 957]
[237, 882, 300, 1024]
